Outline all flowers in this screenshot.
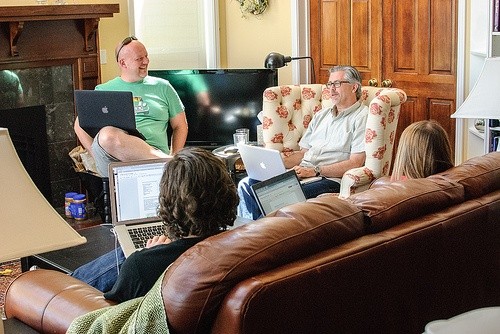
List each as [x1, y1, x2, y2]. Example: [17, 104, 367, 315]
[236, 0, 269, 20]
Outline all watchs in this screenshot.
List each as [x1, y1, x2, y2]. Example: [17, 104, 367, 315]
[314, 166, 321, 177]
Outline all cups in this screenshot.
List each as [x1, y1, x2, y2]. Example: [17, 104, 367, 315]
[236, 128, 249, 144]
[234, 133, 247, 148]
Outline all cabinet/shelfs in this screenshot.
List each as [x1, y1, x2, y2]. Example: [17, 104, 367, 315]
[466, 0, 500, 154]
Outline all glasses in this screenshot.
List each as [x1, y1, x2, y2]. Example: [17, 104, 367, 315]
[117, 36, 137, 62]
[326, 81, 350, 88]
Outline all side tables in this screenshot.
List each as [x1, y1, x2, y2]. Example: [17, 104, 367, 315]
[213, 142, 263, 186]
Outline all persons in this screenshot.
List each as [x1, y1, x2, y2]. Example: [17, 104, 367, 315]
[237, 65, 369, 221]
[390, 121, 453, 180]
[73, 36, 188, 176]
[29, 148, 241, 304]
[0, 69, 24, 110]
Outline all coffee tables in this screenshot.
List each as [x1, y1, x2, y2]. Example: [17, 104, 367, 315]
[21, 223, 120, 275]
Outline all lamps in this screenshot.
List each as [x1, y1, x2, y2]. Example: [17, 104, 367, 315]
[450, 57, 500, 119]
[264, 53, 317, 84]
[0, 127, 87, 263]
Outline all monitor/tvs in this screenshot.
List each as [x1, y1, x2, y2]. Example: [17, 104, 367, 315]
[250, 169, 308, 217]
[147, 68, 278, 149]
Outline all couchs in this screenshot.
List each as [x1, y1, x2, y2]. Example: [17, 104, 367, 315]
[3, 152, 500, 334]
[236, 83, 408, 218]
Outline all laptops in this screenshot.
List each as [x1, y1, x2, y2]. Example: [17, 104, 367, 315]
[237, 143, 322, 185]
[109, 158, 175, 260]
[75, 89, 147, 142]
